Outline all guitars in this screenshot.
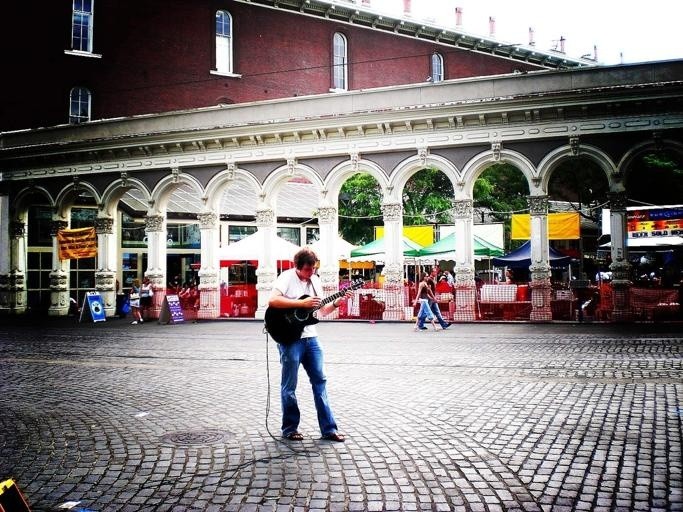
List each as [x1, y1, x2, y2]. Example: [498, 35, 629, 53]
[265, 278, 369, 347]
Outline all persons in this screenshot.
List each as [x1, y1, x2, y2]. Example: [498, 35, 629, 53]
[129, 273, 199, 324]
[413, 268, 513, 331]
[268, 248, 356, 442]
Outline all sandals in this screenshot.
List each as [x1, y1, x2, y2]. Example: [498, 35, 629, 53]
[283, 431, 304, 441]
[323, 432, 345, 442]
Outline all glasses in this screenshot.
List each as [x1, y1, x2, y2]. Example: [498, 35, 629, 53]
[424, 276, 429, 278]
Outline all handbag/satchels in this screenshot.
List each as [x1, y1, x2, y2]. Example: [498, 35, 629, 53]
[130, 292, 139, 299]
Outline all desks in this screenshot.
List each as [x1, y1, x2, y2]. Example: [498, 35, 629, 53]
[474, 298, 579, 320]
[339, 295, 385, 320]
[410, 296, 454, 321]
[221, 295, 254, 318]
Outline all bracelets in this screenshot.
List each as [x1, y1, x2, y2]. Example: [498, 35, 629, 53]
[333, 300, 338, 308]
